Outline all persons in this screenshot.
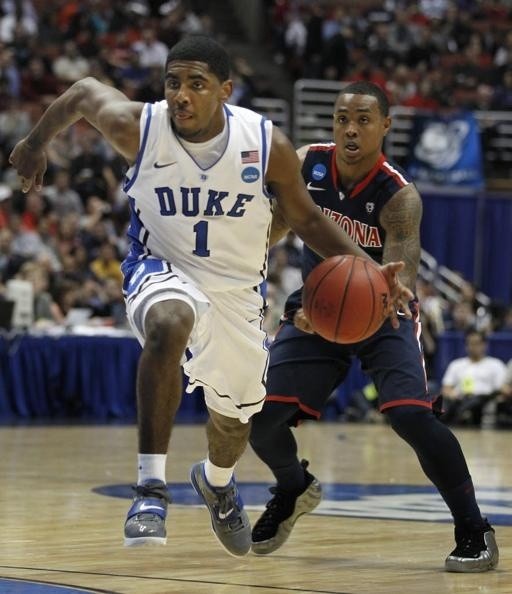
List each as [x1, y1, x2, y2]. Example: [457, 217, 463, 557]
[345, 383, 390, 425]
[8, 35, 415, 559]
[0, 0, 512, 334]
[416, 282, 440, 393]
[245, 75, 501, 574]
[437, 333, 511, 431]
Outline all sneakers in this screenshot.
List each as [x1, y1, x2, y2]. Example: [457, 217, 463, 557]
[123, 480, 169, 547]
[446, 516, 498, 574]
[189, 457, 251, 556]
[252, 458, 323, 555]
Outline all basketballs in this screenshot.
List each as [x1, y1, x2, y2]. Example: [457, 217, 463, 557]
[302, 255, 391, 345]
